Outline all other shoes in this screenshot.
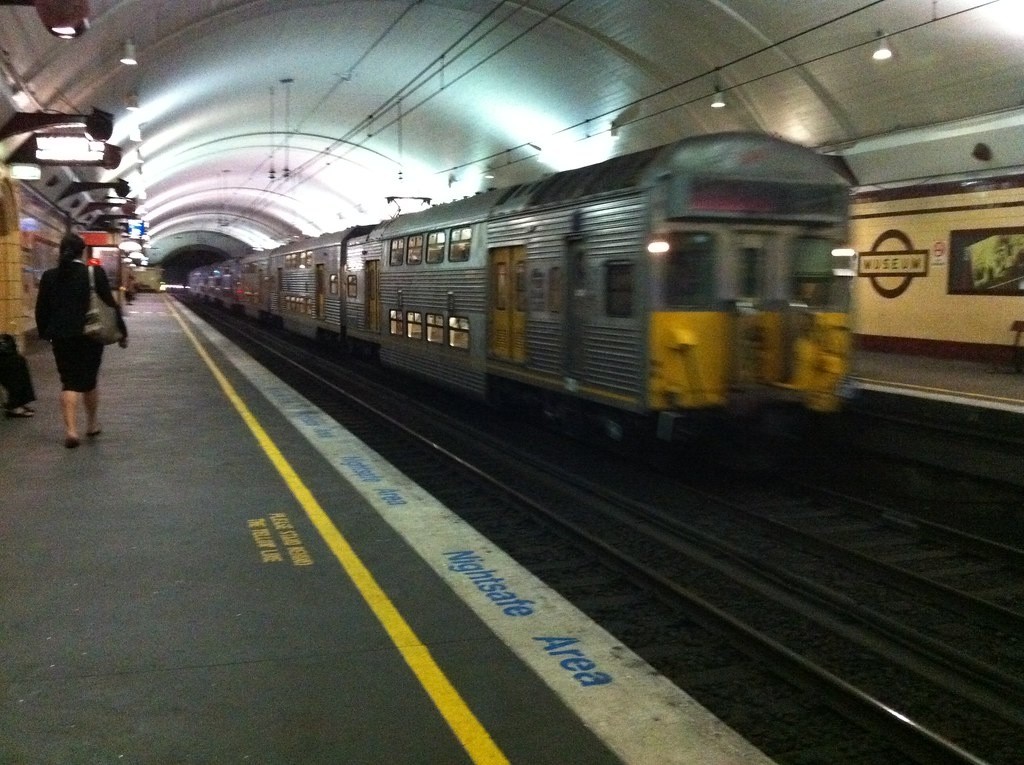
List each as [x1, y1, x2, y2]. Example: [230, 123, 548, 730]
[6, 403, 37, 419]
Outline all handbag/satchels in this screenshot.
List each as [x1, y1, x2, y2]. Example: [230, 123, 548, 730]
[83, 263, 124, 345]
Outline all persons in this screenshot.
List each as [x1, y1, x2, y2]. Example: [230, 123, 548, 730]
[0, 334, 37, 420]
[35, 233, 128, 450]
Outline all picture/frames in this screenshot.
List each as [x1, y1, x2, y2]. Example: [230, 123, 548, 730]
[946, 226, 1024, 297]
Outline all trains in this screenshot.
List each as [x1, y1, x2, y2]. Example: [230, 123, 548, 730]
[186, 130, 856, 453]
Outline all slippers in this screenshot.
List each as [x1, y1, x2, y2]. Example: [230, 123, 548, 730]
[64, 438, 80, 450]
[87, 431, 100, 437]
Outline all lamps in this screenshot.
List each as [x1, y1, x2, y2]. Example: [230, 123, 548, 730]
[125, 93, 139, 110]
[710, 67, 726, 108]
[611, 126, 618, 137]
[873, 29, 891, 60]
[119, 37, 137, 65]
